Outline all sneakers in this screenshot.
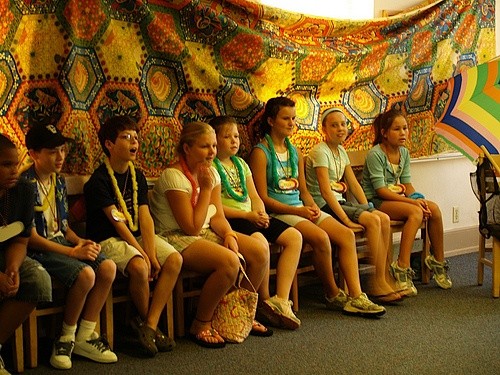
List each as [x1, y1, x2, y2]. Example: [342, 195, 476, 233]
[258, 307, 280, 327]
[425, 255, 452, 289]
[344, 292, 386, 316]
[73, 332, 118, 363]
[49, 335, 75, 369]
[325, 288, 349, 309]
[389, 261, 418, 296]
[263, 295, 301, 329]
[0, 344, 11, 375]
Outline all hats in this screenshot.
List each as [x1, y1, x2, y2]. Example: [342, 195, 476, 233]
[481, 145, 500, 177]
[25, 123, 73, 151]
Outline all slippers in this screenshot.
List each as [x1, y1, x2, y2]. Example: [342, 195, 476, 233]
[395, 288, 414, 296]
[367, 292, 401, 303]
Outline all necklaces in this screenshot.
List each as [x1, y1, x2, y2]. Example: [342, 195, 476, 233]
[35, 172, 56, 211]
[265, 134, 297, 187]
[213, 156, 248, 202]
[103, 156, 139, 232]
[178, 154, 196, 208]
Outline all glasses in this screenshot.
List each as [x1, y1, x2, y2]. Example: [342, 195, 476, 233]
[118, 133, 139, 141]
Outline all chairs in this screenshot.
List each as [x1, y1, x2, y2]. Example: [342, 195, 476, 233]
[14, 166, 432, 371]
[477, 153, 500, 297]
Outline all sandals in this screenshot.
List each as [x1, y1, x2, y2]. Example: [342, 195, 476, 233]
[130, 315, 158, 354]
[250, 322, 273, 337]
[187, 317, 226, 348]
[154, 327, 177, 351]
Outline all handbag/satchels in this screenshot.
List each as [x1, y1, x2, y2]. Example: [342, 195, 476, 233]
[211, 253, 258, 343]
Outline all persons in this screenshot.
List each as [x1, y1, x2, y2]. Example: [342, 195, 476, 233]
[305, 107, 412, 302]
[19, 121, 118, 371]
[0, 134, 52, 375]
[207, 115, 303, 330]
[149, 122, 273, 348]
[360, 109, 452, 296]
[249, 97, 387, 316]
[83, 115, 183, 356]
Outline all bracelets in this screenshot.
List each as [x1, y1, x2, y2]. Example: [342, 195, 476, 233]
[224, 232, 238, 241]
